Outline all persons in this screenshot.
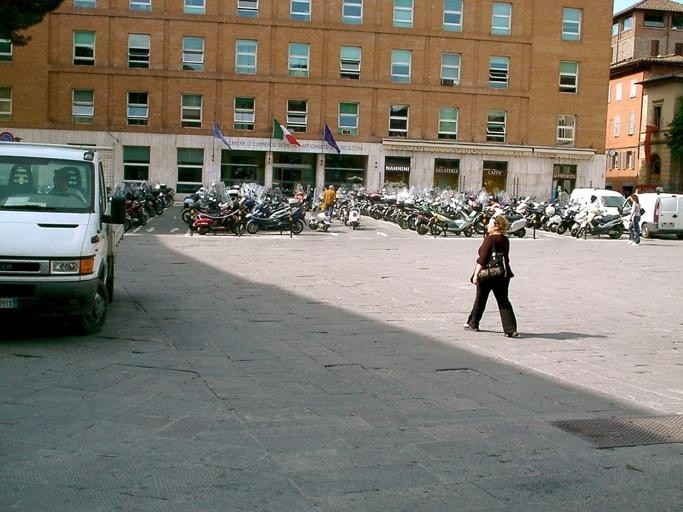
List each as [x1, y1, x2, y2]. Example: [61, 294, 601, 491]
[53, 169, 87, 204]
[294, 184, 336, 223]
[629, 194, 641, 243]
[465, 214, 519, 337]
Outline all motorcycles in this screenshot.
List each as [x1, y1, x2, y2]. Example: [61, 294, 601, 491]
[180, 182, 358, 236]
[104, 184, 174, 232]
[353, 186, 596, 238]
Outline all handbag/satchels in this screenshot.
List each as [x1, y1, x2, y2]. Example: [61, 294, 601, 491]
[478, 252, 509, 283]
[641, 208, 644, 215]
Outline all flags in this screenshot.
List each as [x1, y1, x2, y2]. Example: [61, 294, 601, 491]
[324, 121, 341, 154]
[273, 118, 301, 147]
[213, 120, 231, 149]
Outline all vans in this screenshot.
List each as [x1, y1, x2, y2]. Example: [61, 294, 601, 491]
[570, 189, 682, 237]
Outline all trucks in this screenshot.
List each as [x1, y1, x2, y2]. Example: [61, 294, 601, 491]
[0, 140, 126, 335]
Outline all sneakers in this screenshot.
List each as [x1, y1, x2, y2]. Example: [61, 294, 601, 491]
[464, 323, 478, 332]
[505, 332, 521, 338]
[627, 241, 638, 245]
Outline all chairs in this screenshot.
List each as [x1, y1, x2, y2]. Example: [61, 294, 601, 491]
[9, 165, 86, 196]
